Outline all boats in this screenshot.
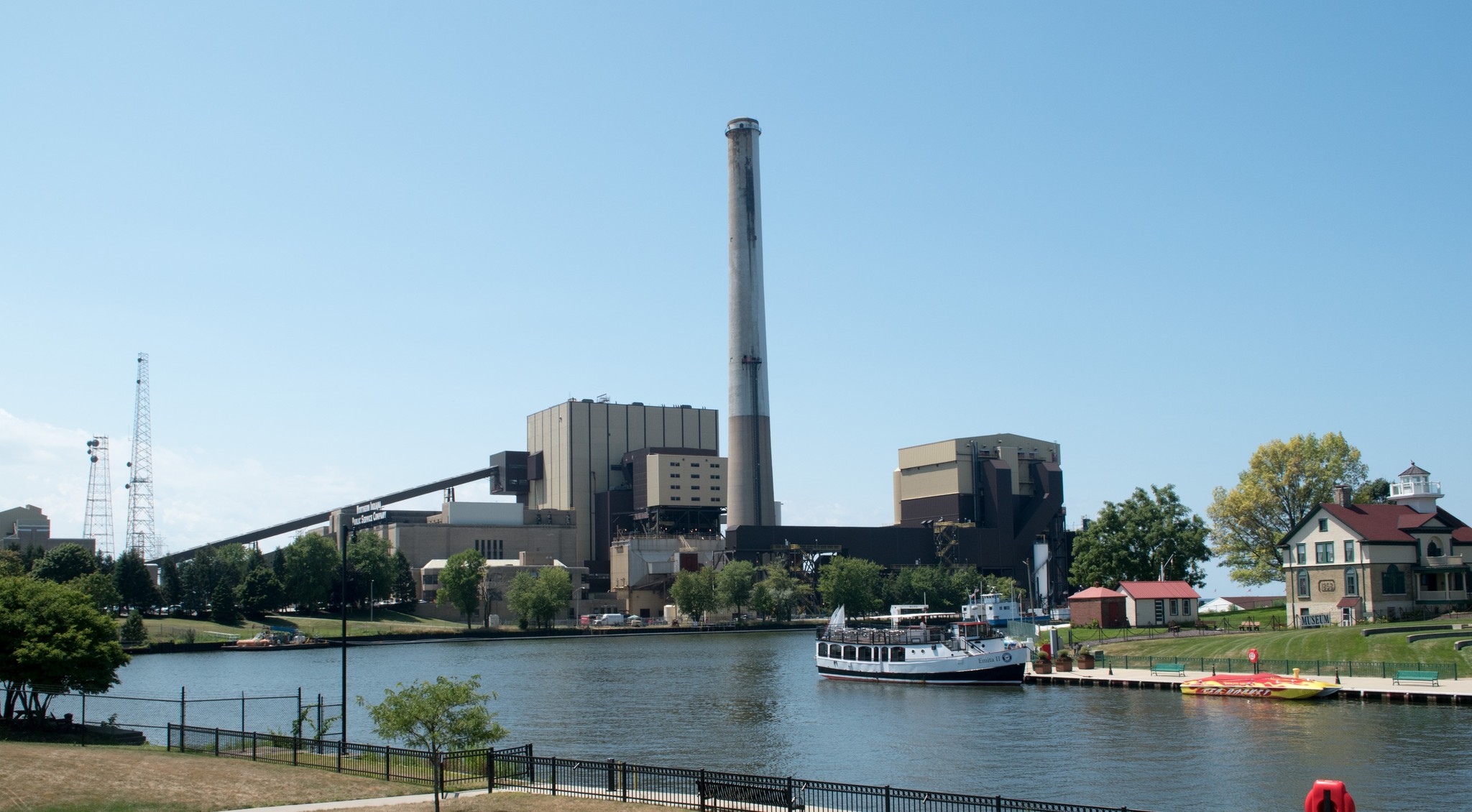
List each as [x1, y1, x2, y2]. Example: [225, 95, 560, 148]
[1050, 608, 1071, 621]
[1179, 668, 1343, 700]
[220, 626, 330, 652]
[961, 585, 1051, 628]
[815, 592, 1027, 686]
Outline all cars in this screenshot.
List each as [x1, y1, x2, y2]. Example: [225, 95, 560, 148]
[103, 606, 135, 613]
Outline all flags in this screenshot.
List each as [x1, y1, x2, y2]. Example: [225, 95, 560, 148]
[971, 588, 979, 599]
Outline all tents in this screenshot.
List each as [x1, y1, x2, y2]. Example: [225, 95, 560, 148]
[627, 615, 641, 623]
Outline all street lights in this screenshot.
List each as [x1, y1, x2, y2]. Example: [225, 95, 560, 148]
[1052, 581, 1054, 601]
[567, 586, 586, 628]
[371, 579, 374, 621]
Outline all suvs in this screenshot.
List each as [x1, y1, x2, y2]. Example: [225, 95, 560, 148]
[161, 604, 182, 613]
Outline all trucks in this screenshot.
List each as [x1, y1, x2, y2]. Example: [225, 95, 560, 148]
[592, 614, 623, 626]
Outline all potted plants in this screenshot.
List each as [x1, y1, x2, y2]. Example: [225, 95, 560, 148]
[1034, 652, 1052, 674]
[1077, 646, 1095, 670]
[1054, 649, 1073, 672]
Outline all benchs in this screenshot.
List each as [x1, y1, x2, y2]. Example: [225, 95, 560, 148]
[695, 780, 805, 812]
[1168, 622, 1181, 632]
[1195, 620, 1216, 631]
[1392, 670, 1439, 687]
[1238, 622, 1260, 631]
[1151, 663, 1185, 677]
